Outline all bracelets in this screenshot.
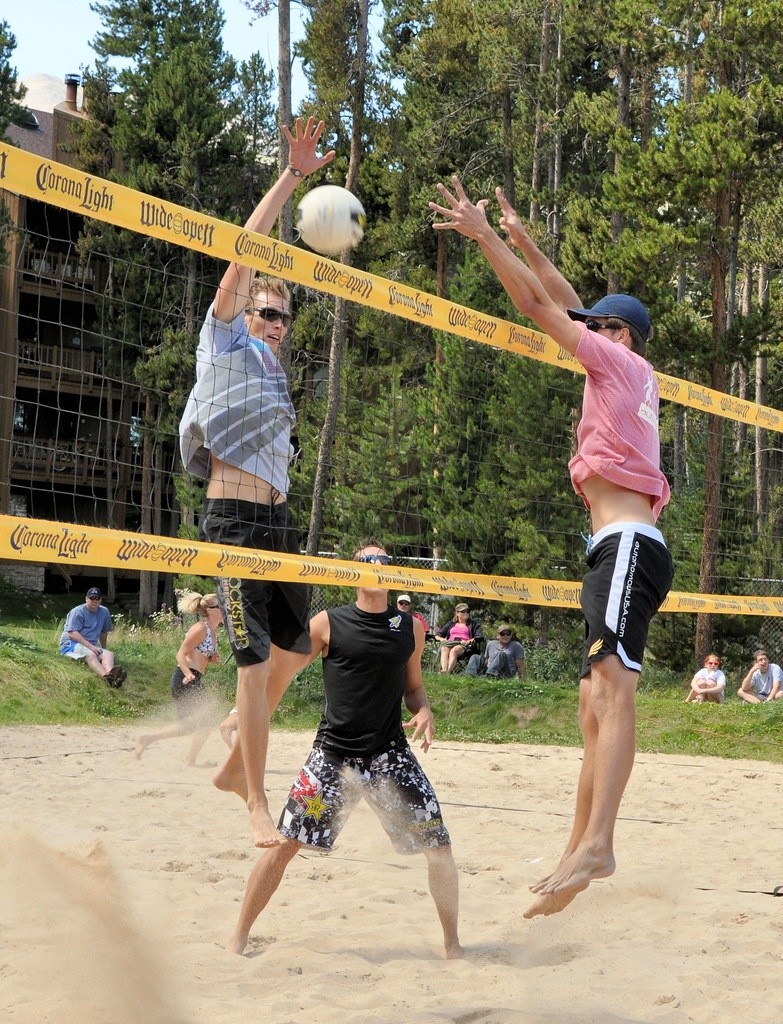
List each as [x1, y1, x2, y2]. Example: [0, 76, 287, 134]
[767, 698, 772, 701]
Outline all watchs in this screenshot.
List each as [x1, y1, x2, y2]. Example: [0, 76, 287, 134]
[287, 165, 306, 180]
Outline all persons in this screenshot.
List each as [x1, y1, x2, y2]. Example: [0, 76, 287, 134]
[179, 115, 337, 847]
[58, 588, 128, 688]
[434, 603, 482, 674]
[396, 594, 429, 638]
[430, 174, 673, 920]
[465, 624, 527, 682]
[737, 650, 783, 705]
[218, 537, 466, 960]
[682, 654, 726, 705]
[135, 590, 224, 768]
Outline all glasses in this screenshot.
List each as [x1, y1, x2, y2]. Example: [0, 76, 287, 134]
[707, 662, 720, 666]
[458, 610, 470, 614]
[399, 601, 410, 605]
[358, 555, 392, 565]
[500, 631, 511, 636]
[585, 319, 624, 333]
[89, 597, 100, 601]
[246, 306, 294, 328]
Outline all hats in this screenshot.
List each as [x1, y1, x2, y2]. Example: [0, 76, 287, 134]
[455, 603, 470, 611]
[87, 587, 102, 598]
[497, 625, 512, 634]
[397, 595, 411, 604]
[567, 295, 651, 342]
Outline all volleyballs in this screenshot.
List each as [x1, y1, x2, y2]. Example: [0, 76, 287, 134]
[293, 183, 369, 259]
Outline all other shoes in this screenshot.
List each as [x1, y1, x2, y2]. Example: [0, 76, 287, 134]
[105, 666, 127, 688]
